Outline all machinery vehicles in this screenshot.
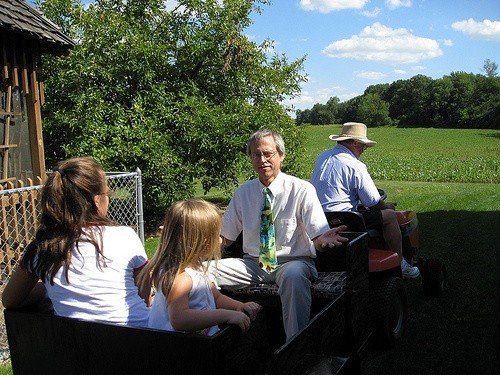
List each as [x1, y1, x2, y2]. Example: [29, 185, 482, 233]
[3, 187, 453, 375]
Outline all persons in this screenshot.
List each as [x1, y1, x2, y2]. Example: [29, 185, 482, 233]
[2, 156, 152, 336]
[205, 127, 350, 345]
[310, 121, 420, 279]
[136, 197, 265, 347]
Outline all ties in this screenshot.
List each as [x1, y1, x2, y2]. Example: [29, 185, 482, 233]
[258, 188, 279, 274]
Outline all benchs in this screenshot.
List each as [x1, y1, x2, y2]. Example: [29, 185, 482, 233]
[3, 298, 286, 375]
[221, 230, 370, 299]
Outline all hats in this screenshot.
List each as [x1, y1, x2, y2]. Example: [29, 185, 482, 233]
[330, 121, 379, 148]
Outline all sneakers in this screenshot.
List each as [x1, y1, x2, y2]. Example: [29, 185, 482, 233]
[401, 261, 419, 280]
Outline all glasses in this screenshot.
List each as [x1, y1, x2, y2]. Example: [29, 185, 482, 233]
[356, 141, 369, 151]
[98, 189, 113, 199]
[250, 150, 278, 159]
